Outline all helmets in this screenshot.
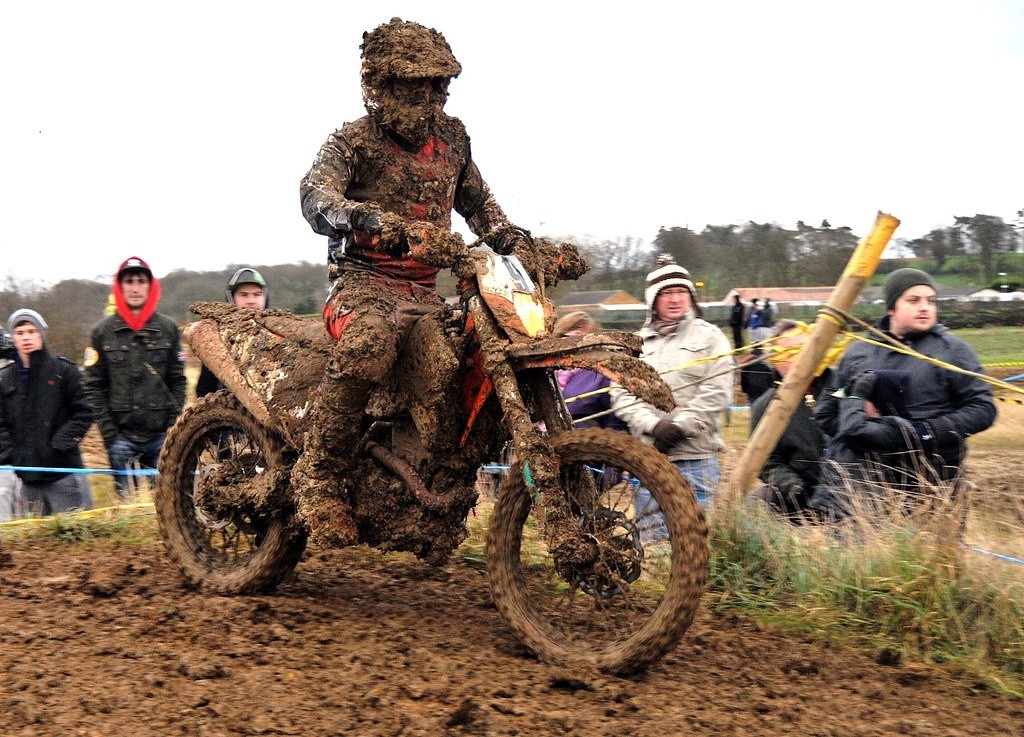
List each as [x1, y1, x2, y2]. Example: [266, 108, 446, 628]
[360, 17, 463, 145]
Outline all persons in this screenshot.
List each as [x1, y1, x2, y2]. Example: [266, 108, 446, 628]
[609, 251, 736, 543]
[728, 294, 773, 350]
[739, 317, 881, 519]
[83, 257, 186, 500]
[195, 267, 269, 461]
[541, 310, 625, 496]
[289, 17, 586, 552]
[1, 308, 95, 514]
[811, 268, 997, 495]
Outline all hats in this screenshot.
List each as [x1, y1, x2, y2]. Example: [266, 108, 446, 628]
[844, 369, 911, 407]
[117, 258, 151, 277]
[640, 252, 704, 327]
[228, 269, 267, 290]
[6, 308, 49, 342]
[882, 267, 938, 308]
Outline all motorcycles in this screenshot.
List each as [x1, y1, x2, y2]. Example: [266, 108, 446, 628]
[151, 213, 710, 684]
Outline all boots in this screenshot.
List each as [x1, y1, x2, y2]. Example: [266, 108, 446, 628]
[290, 398, 364, 553]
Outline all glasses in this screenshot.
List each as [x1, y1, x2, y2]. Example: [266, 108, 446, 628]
[658, 290, 689, 298]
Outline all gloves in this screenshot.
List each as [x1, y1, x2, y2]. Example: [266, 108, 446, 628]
[363, 210, 410, 258]
[107, 437, 138, 463]
[654, 421, 684, 449]
[844, 371, 885, 397]
